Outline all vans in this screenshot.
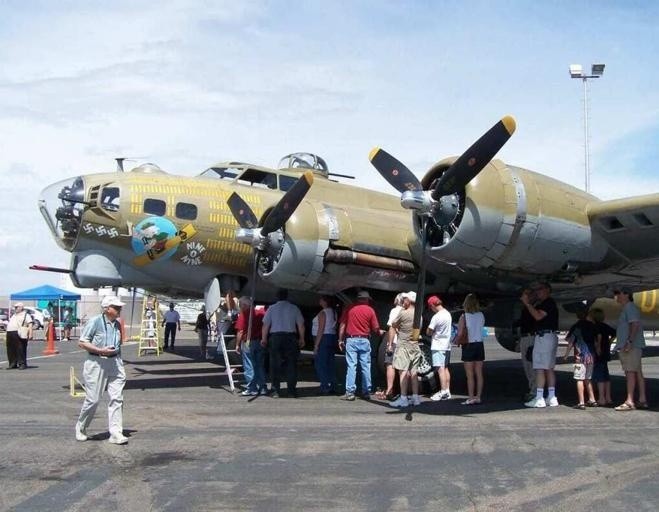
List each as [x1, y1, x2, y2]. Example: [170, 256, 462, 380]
[0, 307, 14, 321]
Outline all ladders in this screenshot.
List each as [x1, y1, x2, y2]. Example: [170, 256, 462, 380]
[138, 297, 164, 357]
[215, 310, 249, 392]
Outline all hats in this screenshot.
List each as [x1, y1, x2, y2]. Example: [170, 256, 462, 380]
[357, 291, 372, 300]
[427, 296, 441, 308]
[14, 302, 24, 307]
[101, 296, 126, 309]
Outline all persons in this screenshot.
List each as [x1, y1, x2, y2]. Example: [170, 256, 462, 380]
[616, 286, 650, 412]
[225, 287, 239, 321]
[5, 300, 32, 371]
[42, 302, 56, 342]
[374, 293, 404, 399]
[520, 283, 558, 409]
[193, 304, 210, 355]
[564, 304, 595, 410]
[518, 284, 541, 404]
[427, 296, 453, 402]
[61, 306, 73, 342]
[75, 293, 129, 446]
[236, 297, 266, 397]
[311, 297, 337, 396]
[161, 303, 181, 353]
[262, 289, 303, 398]
[338, 292, 386, 401]
[591, 308, 616, 407]
[145, 296, 155, 338]
[455, 293, 485, 406]
[389, 291, 421, 407]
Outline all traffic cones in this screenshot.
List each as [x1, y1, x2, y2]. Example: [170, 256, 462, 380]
[41, 322, 60, 355]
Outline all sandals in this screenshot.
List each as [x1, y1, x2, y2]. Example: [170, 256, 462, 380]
[586, 402, 598, 407]
[573, 403, 586, 410]
[635, 401, 648, 409]
[615, 402, 634, 411]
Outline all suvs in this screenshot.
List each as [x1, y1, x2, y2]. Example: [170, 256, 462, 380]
[3, 306, 51, 330]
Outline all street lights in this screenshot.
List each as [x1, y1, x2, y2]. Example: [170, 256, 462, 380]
[569, 63, 606, 193]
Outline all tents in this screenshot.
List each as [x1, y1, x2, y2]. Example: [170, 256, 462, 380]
[8, 284, 81, 343]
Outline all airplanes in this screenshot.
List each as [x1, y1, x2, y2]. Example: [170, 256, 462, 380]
[29, 116, 659, 396]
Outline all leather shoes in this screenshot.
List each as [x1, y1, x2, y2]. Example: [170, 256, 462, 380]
[19, 366, 27, 369]
[6, 366, 17, 370]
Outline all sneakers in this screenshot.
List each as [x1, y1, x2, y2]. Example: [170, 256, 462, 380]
[388, 398, 409, 408]
[409, 398, 420, 406]
[75, 423, 88, 441]
[546, 397, 558, 407]
[430, 391, 452, 401]
[242, 388, 259, 395]
[338, 392, 356, 401]
[524, 398, 546, 408]
[108, 432, 128, 445]
